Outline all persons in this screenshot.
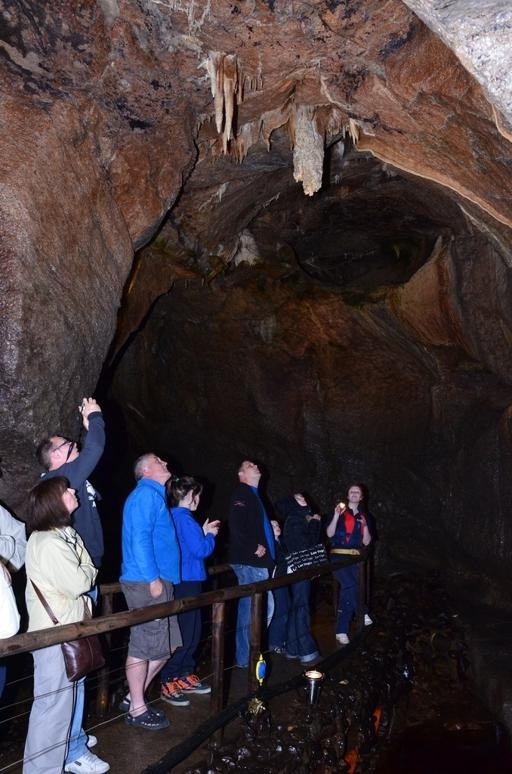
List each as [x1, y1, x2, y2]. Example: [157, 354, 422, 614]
[118, 455, 370, 731]
[20, 479, 98, 774]
[0, 503, 28, 774]
[37, 397, 110, 774]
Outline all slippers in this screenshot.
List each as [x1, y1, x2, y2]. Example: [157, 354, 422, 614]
[117, 697, 164, 718]
[124, 710, 170, 731]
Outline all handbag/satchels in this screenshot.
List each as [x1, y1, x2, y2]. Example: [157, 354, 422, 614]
[59, 635, 106, 682]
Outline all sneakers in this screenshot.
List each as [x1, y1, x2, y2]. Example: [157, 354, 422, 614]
[300, 656, 325, 667]
[174, 674, 212, 694]
[160, 682, 190, 707]
[64, 751, 111, 774]
[85, 732, 98, 746]
[335, 633, 350, 644]
[364, 614, 373, 626]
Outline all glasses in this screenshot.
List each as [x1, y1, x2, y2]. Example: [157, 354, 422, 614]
[53, 440, 70, 453]
